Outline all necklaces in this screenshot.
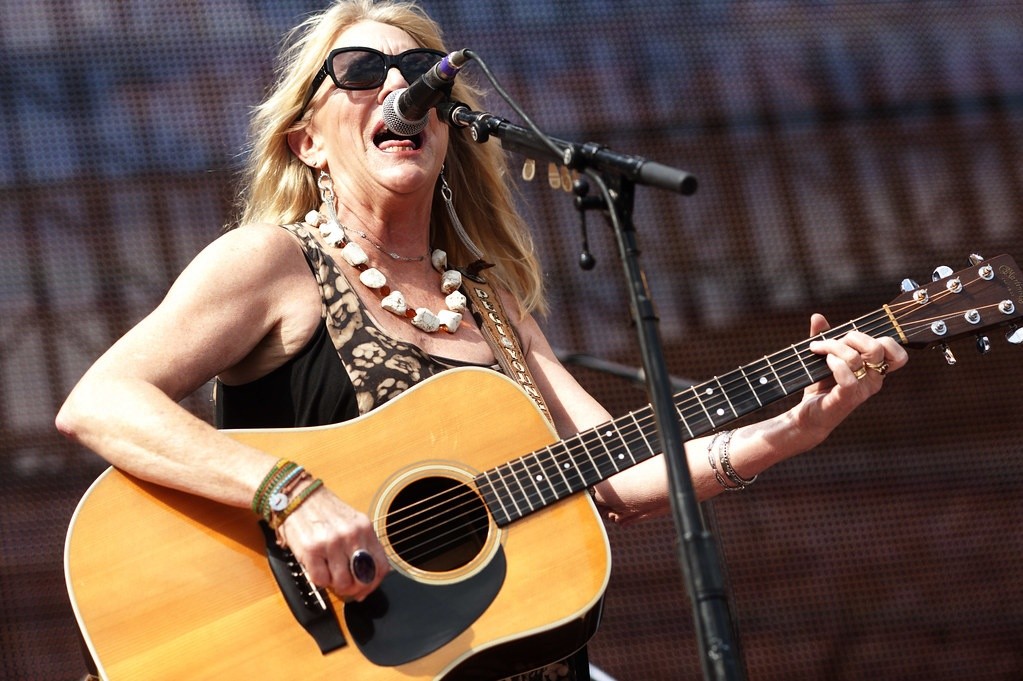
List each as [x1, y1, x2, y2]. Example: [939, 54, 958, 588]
[304, 207, 467, 333]
[319, 211, 433, 262]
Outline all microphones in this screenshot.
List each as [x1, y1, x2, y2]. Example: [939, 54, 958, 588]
[382, 46, 472, 136]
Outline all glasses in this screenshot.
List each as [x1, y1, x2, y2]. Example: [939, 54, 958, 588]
[296, 46, 451, 122]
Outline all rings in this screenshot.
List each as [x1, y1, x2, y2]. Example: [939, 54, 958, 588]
[865, 358, 890, 375]
[853, 360, 867, 380]
[349, 548, 377, 586]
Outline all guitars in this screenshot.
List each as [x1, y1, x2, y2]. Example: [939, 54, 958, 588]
[64, 251, 1023, 681]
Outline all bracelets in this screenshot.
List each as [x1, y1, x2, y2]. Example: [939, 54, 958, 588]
[252, 457, 323, 553]
[707, 427, 758, 494]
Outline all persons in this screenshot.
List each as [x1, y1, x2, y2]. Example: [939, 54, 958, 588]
[53, 6, 909, 681]
